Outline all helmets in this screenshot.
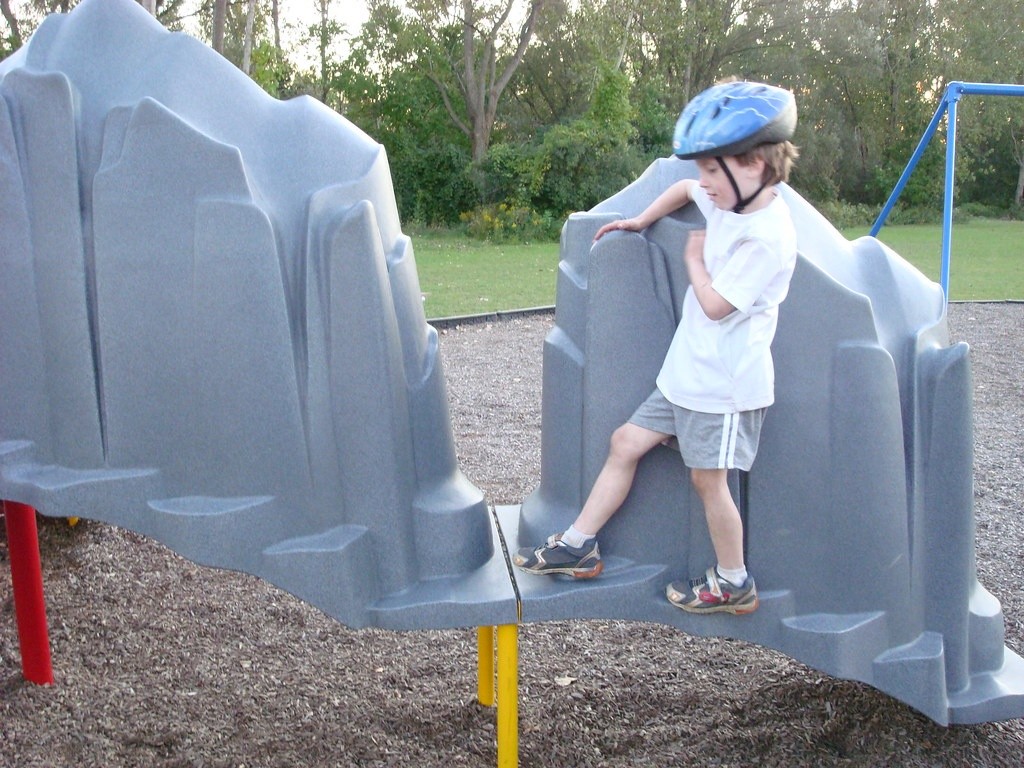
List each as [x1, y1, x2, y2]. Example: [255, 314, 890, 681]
[671, 81, 797, 160]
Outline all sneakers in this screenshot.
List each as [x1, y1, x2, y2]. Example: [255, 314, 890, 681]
[510, 532, 603, 580]
[665, 565, 759, 615]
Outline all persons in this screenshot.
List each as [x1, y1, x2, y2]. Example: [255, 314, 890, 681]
[512, 81, 803, 615]
